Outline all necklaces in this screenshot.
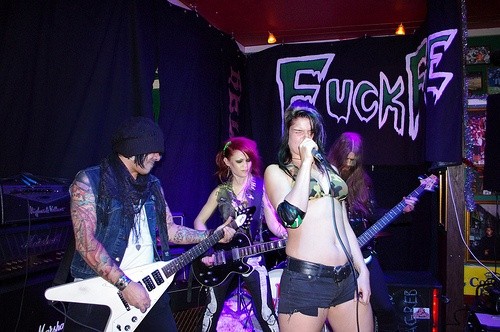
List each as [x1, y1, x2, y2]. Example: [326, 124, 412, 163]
[233, 174, 248, 197]
[292, 158, 315, 164]
[134, 197, 141, 251]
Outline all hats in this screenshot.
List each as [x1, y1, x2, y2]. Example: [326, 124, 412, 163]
[113, 116, 164, 156]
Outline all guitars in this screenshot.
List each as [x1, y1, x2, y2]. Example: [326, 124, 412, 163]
[44, 201, 256, 332]
[188, 232, 289, 287]
[355, 173, 438, 266]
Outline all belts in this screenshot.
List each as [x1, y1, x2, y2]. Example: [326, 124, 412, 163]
[289, 258, 352, 281]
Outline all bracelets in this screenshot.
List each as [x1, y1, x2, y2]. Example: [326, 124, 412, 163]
[208, 229, 215, 237]
[115, 275, 131, 291]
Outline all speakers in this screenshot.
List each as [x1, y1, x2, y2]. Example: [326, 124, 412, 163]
[483, 93, 500, 196]
[168, 285, 211, 332]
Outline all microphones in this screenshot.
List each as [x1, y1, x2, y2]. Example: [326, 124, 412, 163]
[311, 149, 324, 164]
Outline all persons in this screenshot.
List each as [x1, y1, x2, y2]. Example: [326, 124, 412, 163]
[478, 227, 494, 261]
[264, 107, 374, 332]
[327, 132, 419, 332]
[194, 137, 288, 332]
[65, 116, 236, 332]
